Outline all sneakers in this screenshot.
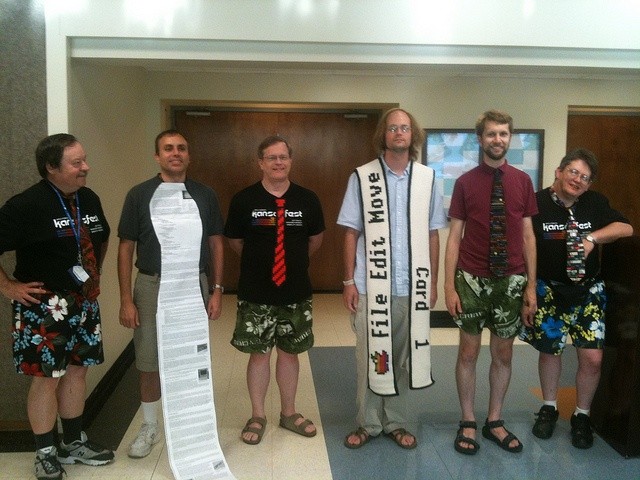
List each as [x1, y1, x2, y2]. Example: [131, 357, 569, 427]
[56, 431, 114, 466]
[570, 411, 593, 449]
[127, 421, 161, 458]
[531, 405, 559, 439]
[34, 446, 68, 480]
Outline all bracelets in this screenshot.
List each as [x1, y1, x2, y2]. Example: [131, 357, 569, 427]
[211, 284, 224, 292]
[342, 279, 354, 286]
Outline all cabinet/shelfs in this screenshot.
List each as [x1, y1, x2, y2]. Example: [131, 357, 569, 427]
[579, 237, 639, 461]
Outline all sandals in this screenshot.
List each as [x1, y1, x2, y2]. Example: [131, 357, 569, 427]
[280, 412, 316, 437]
[482, 417, 523, 453]
[344, 427, 372, 449]
[389, 429, 417, 449]
[454, 421, 480, 455]
[241, 416, 267, 445]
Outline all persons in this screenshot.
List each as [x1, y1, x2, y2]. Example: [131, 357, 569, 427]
[336, 107, 448, 450]
[445, 106, 537, 455]
[117, 128, 226, 460]
[2, 133, 115, 480]
[223, 134, 325, 445]
[518, 149, 635, 449]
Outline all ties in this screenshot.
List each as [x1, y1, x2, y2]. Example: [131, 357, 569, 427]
[489, 169, 508, 277]
[62, 194, 101, 304]
[271, 199, 287, 286]
[550, 187, 586, 284]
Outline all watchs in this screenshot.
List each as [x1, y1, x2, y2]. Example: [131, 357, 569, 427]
[584, 234, 599, 247]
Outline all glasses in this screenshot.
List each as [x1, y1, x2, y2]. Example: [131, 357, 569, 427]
[386, 125, 411, 132]
[566, 166, 591, 182]
